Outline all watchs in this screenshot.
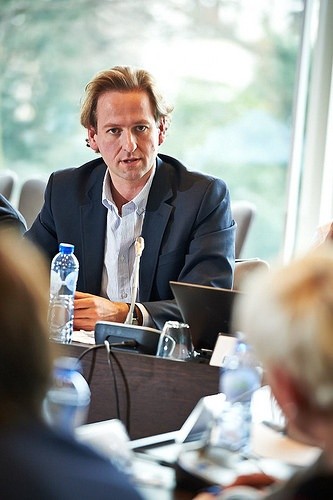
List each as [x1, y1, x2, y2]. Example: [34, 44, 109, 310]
[127, 303, 140, 326]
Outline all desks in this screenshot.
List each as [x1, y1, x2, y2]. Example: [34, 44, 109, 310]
[60, 342, 218, 440]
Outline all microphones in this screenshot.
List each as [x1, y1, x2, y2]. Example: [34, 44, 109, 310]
[94, 237, 176, 357]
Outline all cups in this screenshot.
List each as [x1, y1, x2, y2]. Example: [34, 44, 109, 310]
[156, 321, 194, 362]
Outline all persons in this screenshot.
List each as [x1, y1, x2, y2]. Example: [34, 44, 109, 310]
[22, 66, 236, 331]
[0, 228, 333, 500]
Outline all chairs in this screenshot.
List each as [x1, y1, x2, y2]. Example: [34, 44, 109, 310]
[18, 178, 255, 261]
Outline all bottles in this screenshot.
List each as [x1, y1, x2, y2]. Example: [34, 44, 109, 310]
[47, 243, 79, 344]
[43, 356, 91, 434]
[210, 333, 263, 454]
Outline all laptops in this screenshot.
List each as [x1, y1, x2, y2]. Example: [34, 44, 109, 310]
[169, 281, 241, 359]
